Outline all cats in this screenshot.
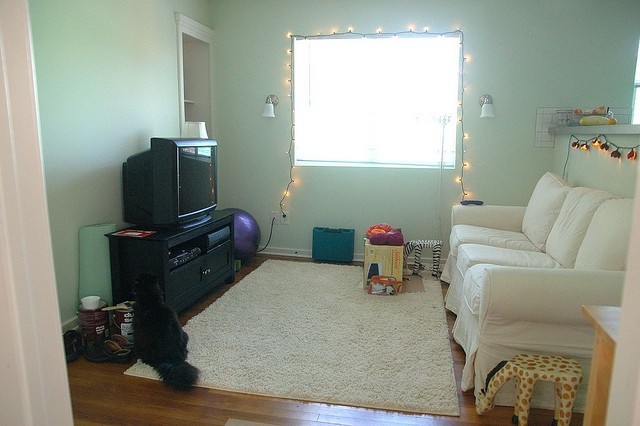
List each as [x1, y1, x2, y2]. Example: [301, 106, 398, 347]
[132, 279, 200, 391]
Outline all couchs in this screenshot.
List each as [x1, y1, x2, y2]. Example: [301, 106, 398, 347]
[441, 181, 640, 417]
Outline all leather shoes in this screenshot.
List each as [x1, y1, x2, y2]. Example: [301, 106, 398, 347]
[63, 329, 82, 362]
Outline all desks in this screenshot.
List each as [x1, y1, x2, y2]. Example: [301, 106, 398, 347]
[582, 304, 623, 426]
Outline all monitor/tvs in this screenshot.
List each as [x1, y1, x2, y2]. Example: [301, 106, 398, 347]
[121, 137, 219, 233]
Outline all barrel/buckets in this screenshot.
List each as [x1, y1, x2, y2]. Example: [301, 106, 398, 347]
[112, 300, 136, 344]
[76, 300, 108, 355]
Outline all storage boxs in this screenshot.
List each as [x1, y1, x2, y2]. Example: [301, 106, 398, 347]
[363, 237, 403, 293]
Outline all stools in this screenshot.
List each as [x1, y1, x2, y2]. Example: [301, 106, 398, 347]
[402, 239, 442, 280]
[476, 354, 580, 425]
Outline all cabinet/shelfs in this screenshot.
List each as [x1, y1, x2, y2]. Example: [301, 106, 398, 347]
[105, 208, 238, 315]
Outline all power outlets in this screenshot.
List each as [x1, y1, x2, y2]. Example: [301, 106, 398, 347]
[280, 212, 289, 224]
[270, 211, 279, 225]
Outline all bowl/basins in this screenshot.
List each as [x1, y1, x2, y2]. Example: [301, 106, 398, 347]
[81, 296, 100, 310]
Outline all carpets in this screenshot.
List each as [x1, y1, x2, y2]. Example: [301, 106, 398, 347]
[125, 259, 463, 419]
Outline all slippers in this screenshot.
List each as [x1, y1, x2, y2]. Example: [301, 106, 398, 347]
[108, 334, 135, 351]
[83, 340, 131, 364]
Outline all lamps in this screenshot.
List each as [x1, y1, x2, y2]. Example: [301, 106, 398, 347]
[261, 95, 278, 117]
[478, 95, 495, 120]
